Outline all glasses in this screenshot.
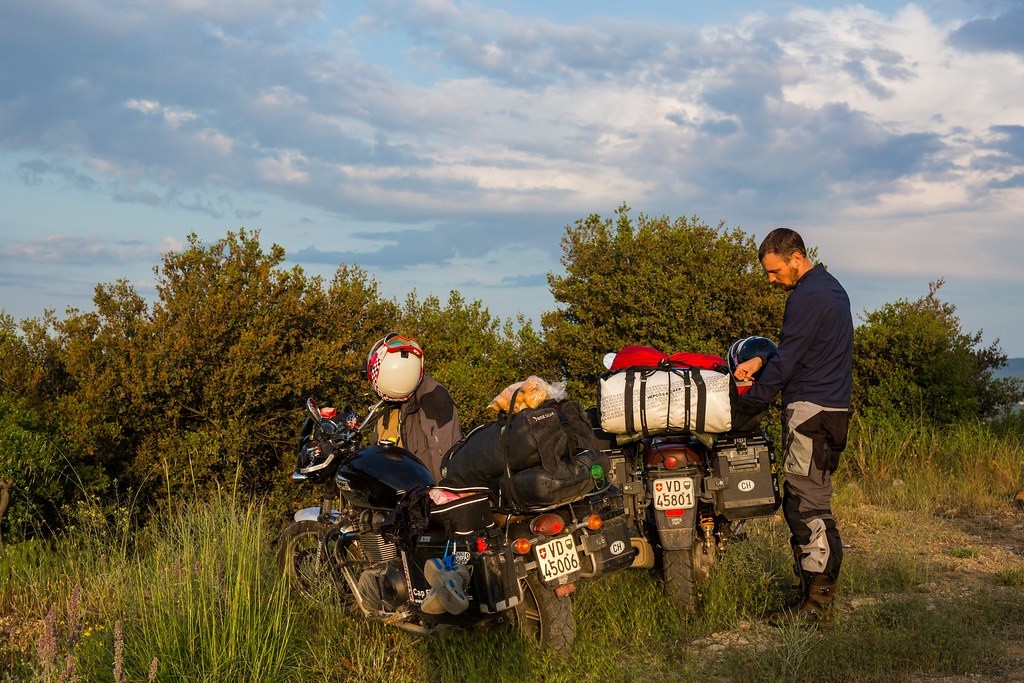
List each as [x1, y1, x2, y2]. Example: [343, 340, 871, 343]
[373, 332, 424, 401]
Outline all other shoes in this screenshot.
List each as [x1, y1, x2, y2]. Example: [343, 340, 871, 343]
[421, 555, 473, 616]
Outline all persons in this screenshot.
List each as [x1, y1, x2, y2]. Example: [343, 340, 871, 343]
[375, 374, 460, 485]
[733, 228, 853, 630]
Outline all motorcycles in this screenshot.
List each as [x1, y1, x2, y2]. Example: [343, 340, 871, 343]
[582, 350, 783, 624]
[280, 391, 636, 672]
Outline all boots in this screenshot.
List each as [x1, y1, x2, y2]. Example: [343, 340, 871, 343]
[769, 574, 836, 631]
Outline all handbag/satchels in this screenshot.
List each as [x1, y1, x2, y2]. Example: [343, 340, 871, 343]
[599, 359, 738, 434]
[440, 399, 594, 488]
[379, 483, 495, 551]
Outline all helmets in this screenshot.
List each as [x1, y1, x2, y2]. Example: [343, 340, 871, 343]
[726, 336, 778, 383]
[367, 332, 426, 402]
